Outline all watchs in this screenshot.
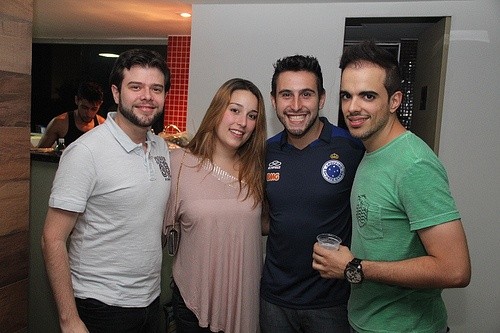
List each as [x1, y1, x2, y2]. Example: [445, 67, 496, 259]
[344, 258, 364, 285]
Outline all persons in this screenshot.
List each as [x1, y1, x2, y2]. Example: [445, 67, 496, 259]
[41, 49, 171, 333]
[312, 43, 472, 333]
[161, 79, 271, 333]
[36, 83, 106, 147]
[255, 55, 365, 333]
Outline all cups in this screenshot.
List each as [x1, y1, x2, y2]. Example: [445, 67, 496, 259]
[316, 233, 342, 274]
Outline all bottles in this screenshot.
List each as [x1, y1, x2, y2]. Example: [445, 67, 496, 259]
[54, 138, 66, 156]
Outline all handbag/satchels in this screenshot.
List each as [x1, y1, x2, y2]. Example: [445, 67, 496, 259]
[166, 150, 187, 257]
